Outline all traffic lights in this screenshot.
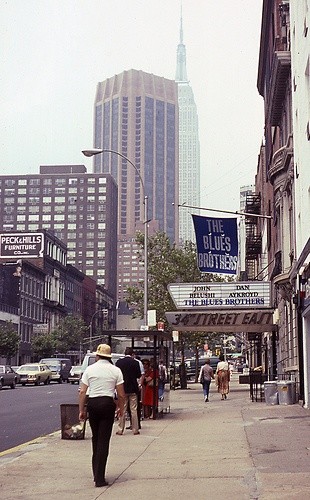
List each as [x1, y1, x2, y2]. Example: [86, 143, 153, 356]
[103, 309, 108, 320]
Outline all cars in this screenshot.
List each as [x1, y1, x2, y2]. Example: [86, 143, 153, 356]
[15, 363, 52, 386]
[0, 365, 20, 390]
[67, 366, 82, 385]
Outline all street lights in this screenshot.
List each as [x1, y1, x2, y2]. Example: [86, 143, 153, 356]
[82, 148, 151, 349]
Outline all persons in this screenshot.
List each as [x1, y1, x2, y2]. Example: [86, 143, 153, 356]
[115, 348, 142, 435]
[229, 363, 233, 376]
[132, 348, 157, 420]
[198, 359, 215, 402]
[216, 355, 230, 400]
[79, 344, 125, 488]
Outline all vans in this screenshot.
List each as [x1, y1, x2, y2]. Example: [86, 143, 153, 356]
[37, 358, 71, 384]
[182, 358, 221, 381]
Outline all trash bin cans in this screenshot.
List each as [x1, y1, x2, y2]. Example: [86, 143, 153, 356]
[278, 380, 297, 404]
[59, 402, 88, 440]
[263, 380, 278, 405]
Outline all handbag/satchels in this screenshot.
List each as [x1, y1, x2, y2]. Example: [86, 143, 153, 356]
[200, 370, 204, 384]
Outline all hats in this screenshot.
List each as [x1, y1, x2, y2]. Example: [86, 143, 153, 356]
[92, 344, 113, 358]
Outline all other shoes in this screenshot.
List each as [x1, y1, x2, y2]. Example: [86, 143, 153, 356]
[148, 378, 158, 386]
[95, 481, 108, 486]
